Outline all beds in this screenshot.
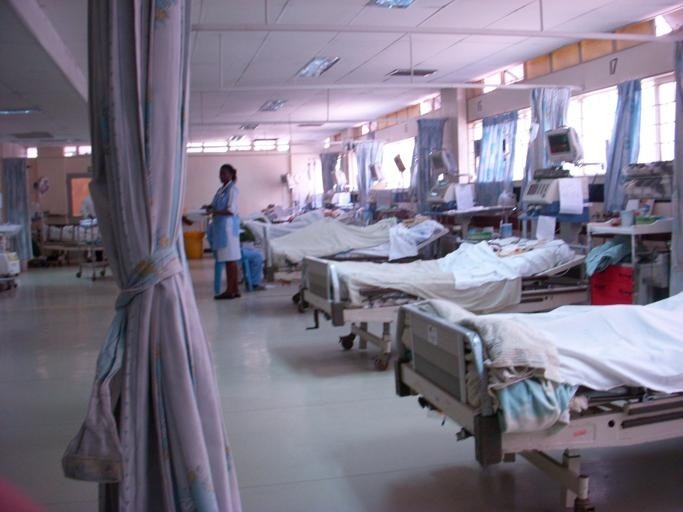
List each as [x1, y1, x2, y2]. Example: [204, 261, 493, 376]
[393, 300, 682, 511]
[302, 234, 601, 372]
[242, 207, 448, 289]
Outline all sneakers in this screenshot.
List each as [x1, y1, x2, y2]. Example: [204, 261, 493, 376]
[214, 292, 241, 300]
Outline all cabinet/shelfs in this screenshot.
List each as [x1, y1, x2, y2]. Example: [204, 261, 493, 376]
[73, 223, 109, 282]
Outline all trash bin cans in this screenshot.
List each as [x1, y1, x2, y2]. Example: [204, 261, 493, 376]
[183, 230, 206, 260]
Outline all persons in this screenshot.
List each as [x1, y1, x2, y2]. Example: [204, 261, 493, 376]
[385, 210, 417, 225]
[238, 246, 267, 291]
[321, 206, 343, 219]
[198, 164, 241, 300]
[81, 183, 103, 262]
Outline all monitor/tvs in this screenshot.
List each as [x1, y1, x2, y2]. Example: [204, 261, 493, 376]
[545, 127, 584, 161]
[330, 170, 340, 185]
[369, 163, 380, 181]
[431, 151, 450, 170]
